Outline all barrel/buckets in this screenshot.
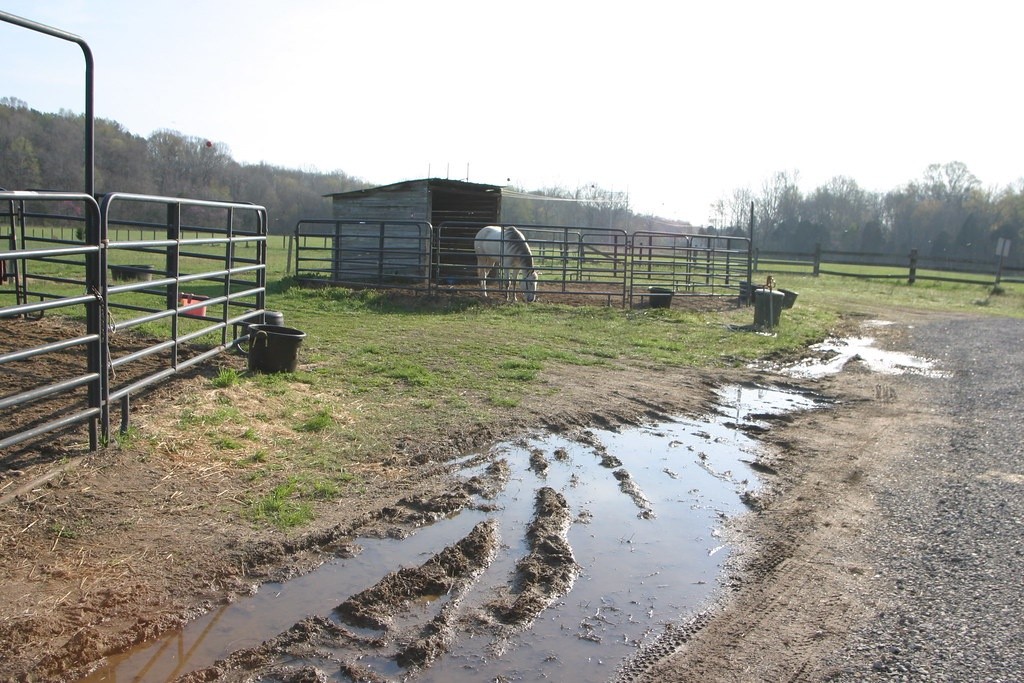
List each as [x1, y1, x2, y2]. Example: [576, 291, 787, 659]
[111, 264, 154, 283]
[247, 324, 307, 374]
[777, 288, 798, 310]
[738, 280, 757, 307]
[179, 294, 208, 317]
[647, 286, 674, 309]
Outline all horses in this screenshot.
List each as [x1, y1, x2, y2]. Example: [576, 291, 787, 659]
[474, 226, 538, 302]
